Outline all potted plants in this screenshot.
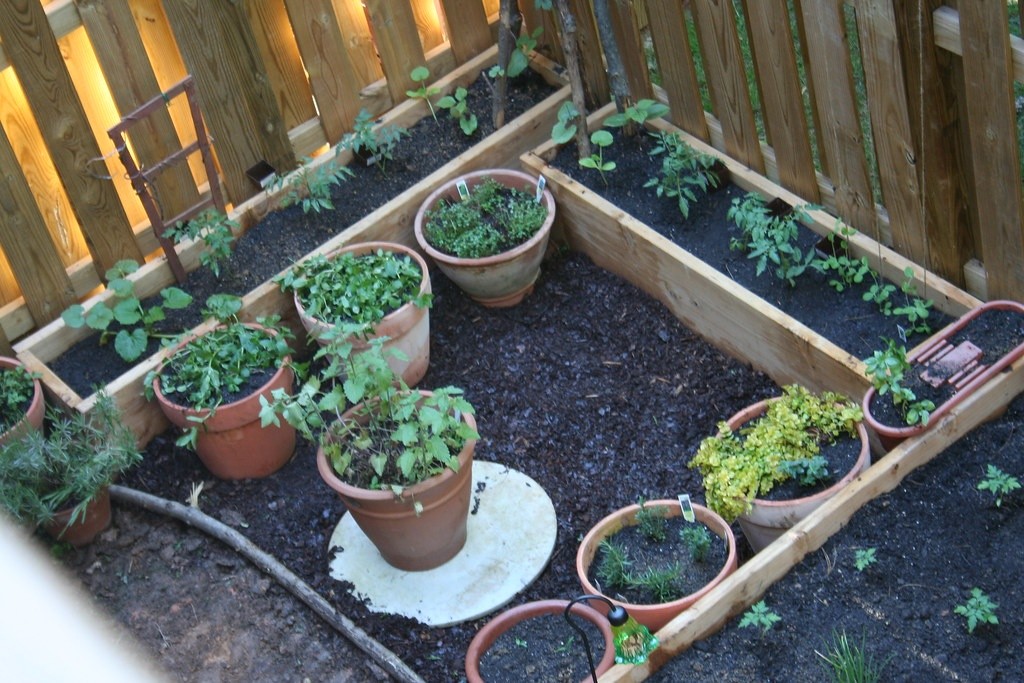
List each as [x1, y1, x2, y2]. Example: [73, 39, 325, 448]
[0, 40, 1022, 683]
